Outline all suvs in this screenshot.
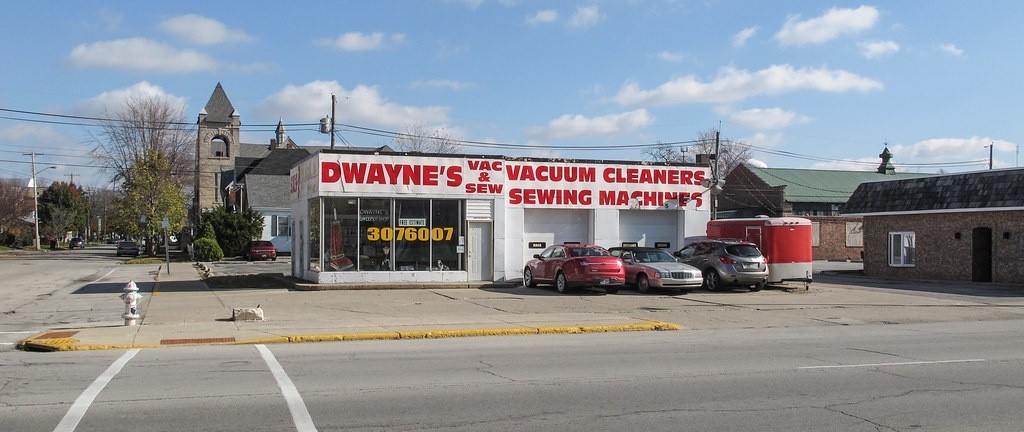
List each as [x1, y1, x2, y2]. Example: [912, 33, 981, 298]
[671, 240, 769, 292]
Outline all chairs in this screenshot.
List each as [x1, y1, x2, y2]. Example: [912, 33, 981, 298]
[623, 254, 631, 263]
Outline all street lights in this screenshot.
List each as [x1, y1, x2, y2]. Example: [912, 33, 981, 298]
[32, 166, 57, 250]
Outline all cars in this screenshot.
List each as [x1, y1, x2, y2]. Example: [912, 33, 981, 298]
[115, 242, 141, 257]
[523, 245, 625, 295]
[606, 246, 704, 295]
[243, 241, 276, 262]
[69, 237, 86, 249]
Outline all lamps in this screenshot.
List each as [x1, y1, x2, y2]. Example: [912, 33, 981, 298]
[954, 232, 960, 243]
[1002, 232, 1009, 242]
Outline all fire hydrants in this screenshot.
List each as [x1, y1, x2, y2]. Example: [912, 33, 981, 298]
[120, 280, 144, 326]
